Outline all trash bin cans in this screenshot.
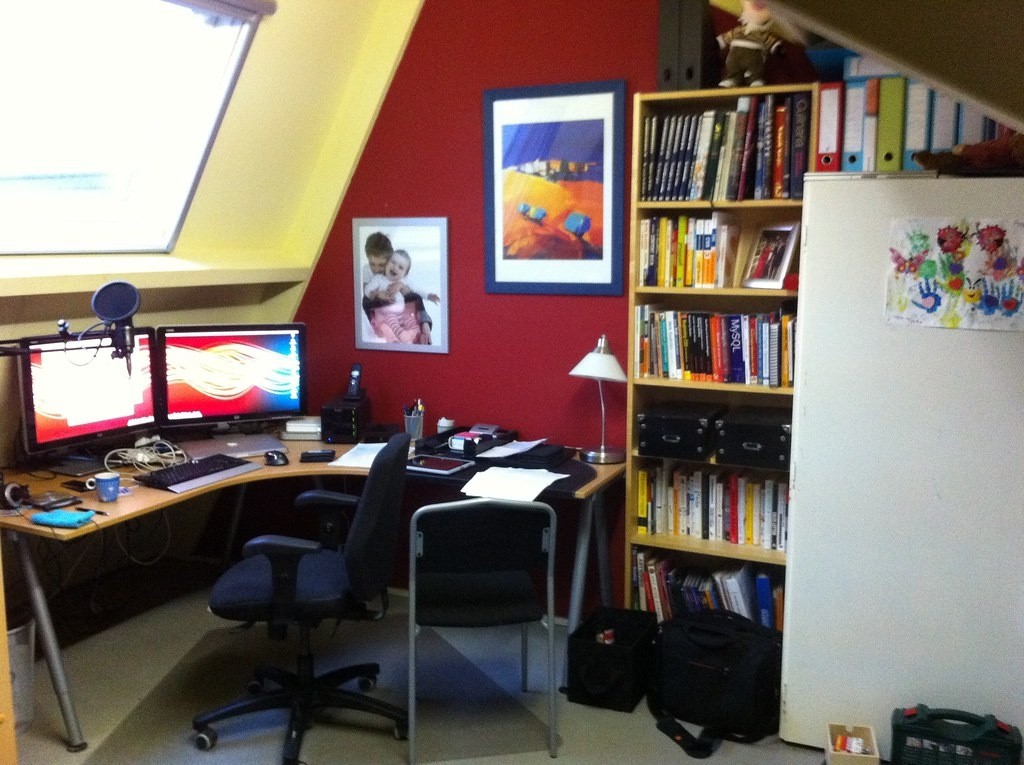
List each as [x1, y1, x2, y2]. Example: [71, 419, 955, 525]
[6, 603, 36, 737]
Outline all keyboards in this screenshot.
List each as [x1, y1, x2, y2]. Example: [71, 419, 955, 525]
[132, 453, 263, 494]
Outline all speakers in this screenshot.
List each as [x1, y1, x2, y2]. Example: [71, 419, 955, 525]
[321, 397, 370, 444]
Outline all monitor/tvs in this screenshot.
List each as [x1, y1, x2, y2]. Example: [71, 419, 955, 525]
[156, 322, 307, 462]
[17, 326, 159, 477]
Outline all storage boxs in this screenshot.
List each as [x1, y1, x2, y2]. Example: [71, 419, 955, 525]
[299, 450, 335, 463]
[279, 430, 323, 441]
[285, 415, 322, 433]
[563, 605, 661, 714]
[365, 424, 398, 442]
[823, 722, 880, 765]
[638, 399, 730, 462]
[716, 404, 792, 470]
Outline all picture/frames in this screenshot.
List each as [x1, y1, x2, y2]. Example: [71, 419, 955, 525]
[351, 217, 451, 355]
[482, 79, 625, 297]
[740, 220, 801, 290]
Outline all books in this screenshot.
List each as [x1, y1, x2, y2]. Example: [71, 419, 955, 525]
[637, 460, 787, 551]
[634, 304, 797, 388]
[631, 546, 784, 631]
[639, 212, 741, 288]
[641, 95, 811, 201]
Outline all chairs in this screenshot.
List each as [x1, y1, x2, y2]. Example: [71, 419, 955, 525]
[405, 496, 559, 765]
[188, 431, 410, 765]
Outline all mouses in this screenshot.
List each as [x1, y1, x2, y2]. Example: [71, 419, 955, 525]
[264, 451, 289, 466]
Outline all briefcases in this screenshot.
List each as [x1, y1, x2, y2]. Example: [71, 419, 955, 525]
[889, 703, 1022, 765]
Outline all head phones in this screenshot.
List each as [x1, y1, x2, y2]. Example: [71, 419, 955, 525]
[0, 482, 30, 509]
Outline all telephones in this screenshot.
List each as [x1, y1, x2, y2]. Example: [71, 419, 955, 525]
[343, 364, 366, 401]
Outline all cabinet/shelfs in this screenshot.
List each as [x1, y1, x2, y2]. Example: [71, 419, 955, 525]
[622, 81, 820, 614]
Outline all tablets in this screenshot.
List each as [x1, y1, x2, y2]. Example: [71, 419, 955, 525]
[406, 454, 476, 475]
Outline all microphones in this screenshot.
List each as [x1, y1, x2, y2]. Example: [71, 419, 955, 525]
[109, 317, 135, 377]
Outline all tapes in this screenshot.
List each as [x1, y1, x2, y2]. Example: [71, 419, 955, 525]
[474, 437, 483, 444]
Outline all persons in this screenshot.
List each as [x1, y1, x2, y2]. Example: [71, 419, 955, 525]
[752, 236, 785, 279]
[362, 231, 439, 345]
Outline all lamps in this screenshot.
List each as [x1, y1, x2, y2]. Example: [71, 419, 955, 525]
[566, 333, 628, 465]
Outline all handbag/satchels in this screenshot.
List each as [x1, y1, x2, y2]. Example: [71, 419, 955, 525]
[644, 607, 781, 760]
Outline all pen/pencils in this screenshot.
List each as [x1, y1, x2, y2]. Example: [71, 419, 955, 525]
[402, 398, 425, 439]
[75, 507, 107, 516]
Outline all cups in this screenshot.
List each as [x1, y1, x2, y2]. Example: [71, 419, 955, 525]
[436, 424, 453, 433]
[404, 415, 423, 441]
[86, 472, 120, 503]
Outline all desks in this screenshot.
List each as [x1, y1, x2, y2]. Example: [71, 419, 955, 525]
[1, 433, 625, 756]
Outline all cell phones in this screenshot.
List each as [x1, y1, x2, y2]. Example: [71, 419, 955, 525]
[62, 481, 94, 491]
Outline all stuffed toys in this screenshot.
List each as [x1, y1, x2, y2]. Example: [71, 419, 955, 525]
[716, 0, 784, 88]
